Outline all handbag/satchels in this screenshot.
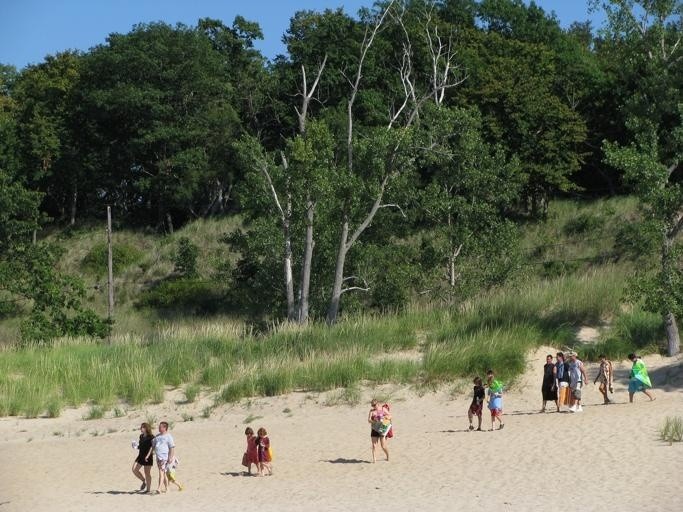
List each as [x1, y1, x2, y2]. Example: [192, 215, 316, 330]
[242, 453, 250, 467]
[266, 447, 273, 461]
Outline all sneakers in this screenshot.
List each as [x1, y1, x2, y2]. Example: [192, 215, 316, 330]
[468, 426, 474, 431]
[500, 424, 504, 429]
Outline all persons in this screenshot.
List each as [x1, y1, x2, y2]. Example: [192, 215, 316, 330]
[538, 351, 588, 414]
[627, 354, 655, 403]
[129, 421, 183, 494]
[241, 426, 260, 476]
[255, 427, 272, 477]
[593, 354, 614, 404]
[368, 399, 392, 464]
[464, 370, 505, 432]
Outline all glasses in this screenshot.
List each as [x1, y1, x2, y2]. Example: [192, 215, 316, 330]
[372, 404, 376, 406]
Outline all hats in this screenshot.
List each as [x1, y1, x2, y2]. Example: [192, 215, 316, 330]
[568, 352, 577, 357]
